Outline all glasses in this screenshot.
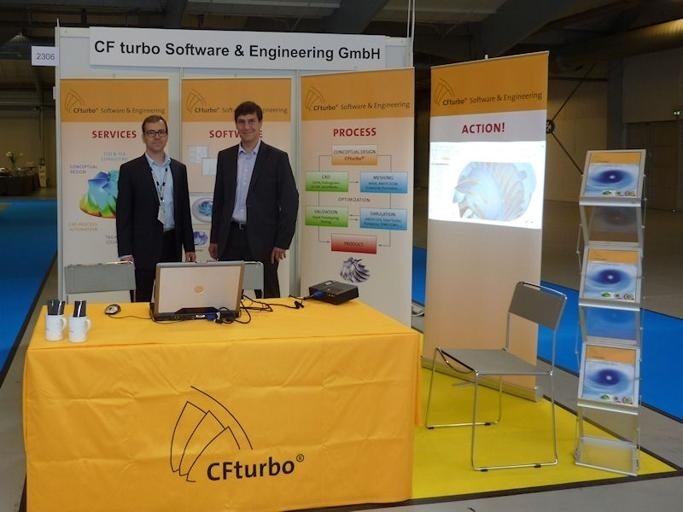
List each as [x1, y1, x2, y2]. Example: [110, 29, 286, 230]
[144, 129, 167, 137]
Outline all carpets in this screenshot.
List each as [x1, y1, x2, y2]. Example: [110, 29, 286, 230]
[409, 327, 680, 503]
[412, 244, 681, 421]
[0, 198, 57, 386]
[394, 359, 680, 504]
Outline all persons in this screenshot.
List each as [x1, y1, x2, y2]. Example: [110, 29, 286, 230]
[116, 115, 196, 302]
[208, 101, 299, 298]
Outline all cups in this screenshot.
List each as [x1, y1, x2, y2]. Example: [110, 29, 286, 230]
[68, 316, 91, 343]
[43, 314, 66, 342]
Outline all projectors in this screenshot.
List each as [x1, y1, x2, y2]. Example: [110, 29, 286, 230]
[309, 280, 359, 305]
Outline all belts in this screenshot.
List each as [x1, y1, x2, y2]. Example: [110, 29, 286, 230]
[231, 221, 247, 232]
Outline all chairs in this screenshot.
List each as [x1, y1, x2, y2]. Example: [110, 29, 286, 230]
[428, 280, 566, 471]
[61, 259, 137, 303]
[239, 259, 263, 299]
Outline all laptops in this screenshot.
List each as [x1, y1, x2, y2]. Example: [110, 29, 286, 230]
[149, 261, 244, 322]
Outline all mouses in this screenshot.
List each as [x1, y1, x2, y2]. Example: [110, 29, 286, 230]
[105, 304, 121, 314]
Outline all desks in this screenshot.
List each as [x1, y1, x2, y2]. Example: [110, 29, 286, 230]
[24, 300, 421, 512]
